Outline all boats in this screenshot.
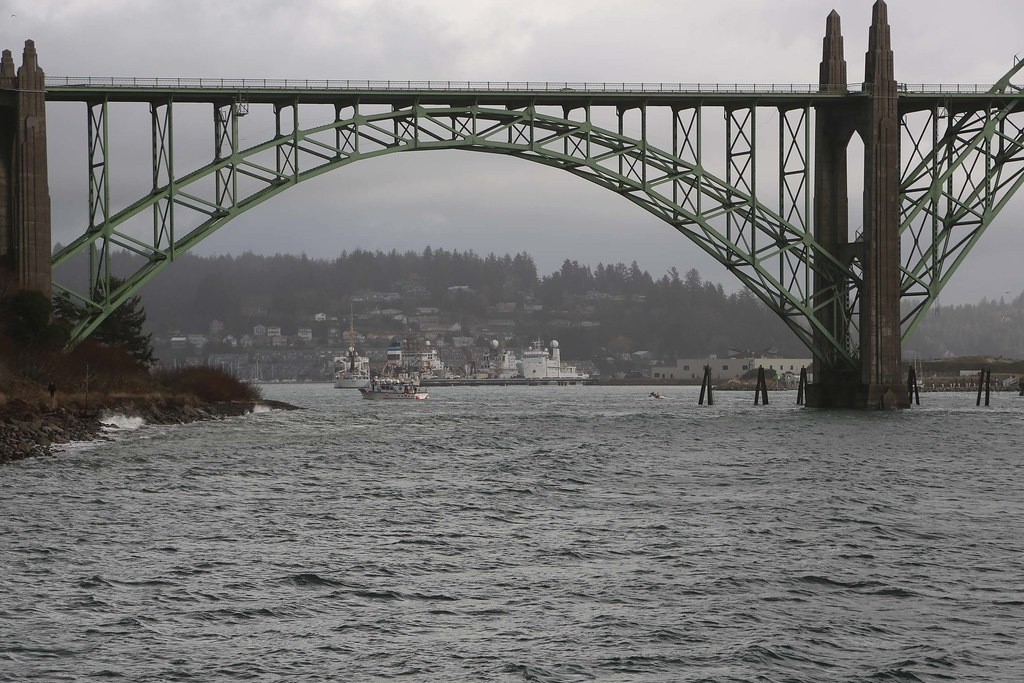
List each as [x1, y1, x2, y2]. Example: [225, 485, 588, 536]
[335, 302, 370, 388]
[359, 359, 429, 399]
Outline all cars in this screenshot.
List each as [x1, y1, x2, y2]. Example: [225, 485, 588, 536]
[444, 372, 460, 379]
[420, 372, 438, 379]
